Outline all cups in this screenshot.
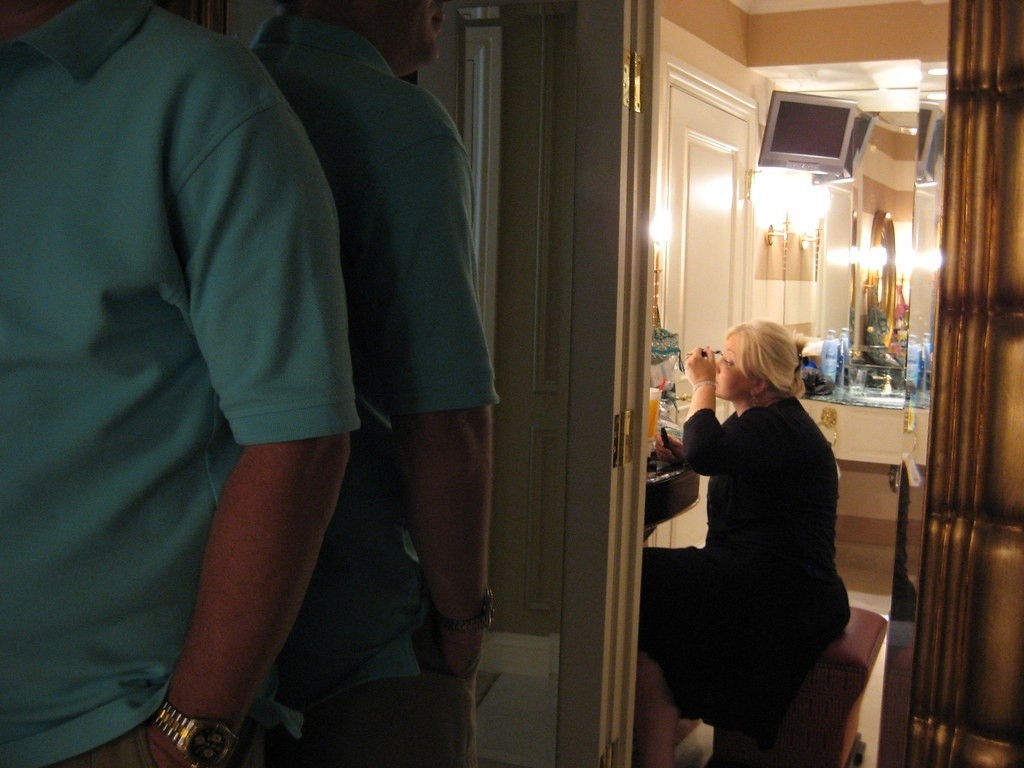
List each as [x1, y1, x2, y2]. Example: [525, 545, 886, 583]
[647, 387, 663, 442]
[848, 369, 867, 395]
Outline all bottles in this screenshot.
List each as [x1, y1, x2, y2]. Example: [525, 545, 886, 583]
[914, 332, 933, 409]
[903, 387, 914, 433]
[838, 328, 849, 365]
[819, 330, 838, 386]
[836, 345, 844, 387]
[905, 335, 920, 388]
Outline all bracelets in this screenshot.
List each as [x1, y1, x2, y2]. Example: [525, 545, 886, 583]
[693, 381, 716, 392]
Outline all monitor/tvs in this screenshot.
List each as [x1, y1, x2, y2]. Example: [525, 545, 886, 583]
[915, 100, 946, 189]
[811, 113, 879, 185]
[757, 90, 857, 176]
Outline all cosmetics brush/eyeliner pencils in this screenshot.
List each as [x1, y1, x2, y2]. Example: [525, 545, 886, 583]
[689, 350, 721, 357]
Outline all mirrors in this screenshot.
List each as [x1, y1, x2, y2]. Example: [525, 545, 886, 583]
[180, 0, 583, 768]
[867, 209, 896, 348]
[848, 111, 919, 370]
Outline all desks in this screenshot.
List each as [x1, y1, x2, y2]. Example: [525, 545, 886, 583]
[726, 383, 905, 466]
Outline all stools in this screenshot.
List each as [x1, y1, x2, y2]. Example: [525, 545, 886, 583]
[703, 605, 889, 768]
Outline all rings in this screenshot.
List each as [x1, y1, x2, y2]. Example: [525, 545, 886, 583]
[685, 354, 690, 359]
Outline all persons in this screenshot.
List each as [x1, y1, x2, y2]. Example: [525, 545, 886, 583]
[634, 319, 851, 768]
[244, 0, 500, 768]
[0, 0, 359, 768]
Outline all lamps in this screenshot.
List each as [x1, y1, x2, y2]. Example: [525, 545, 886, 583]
[763, 208, 825, 285]
[653, 207, 671, 330]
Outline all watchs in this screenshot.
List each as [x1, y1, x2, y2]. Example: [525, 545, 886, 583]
[152, 696, 239, 768]
[427, 586, 494, 633]
